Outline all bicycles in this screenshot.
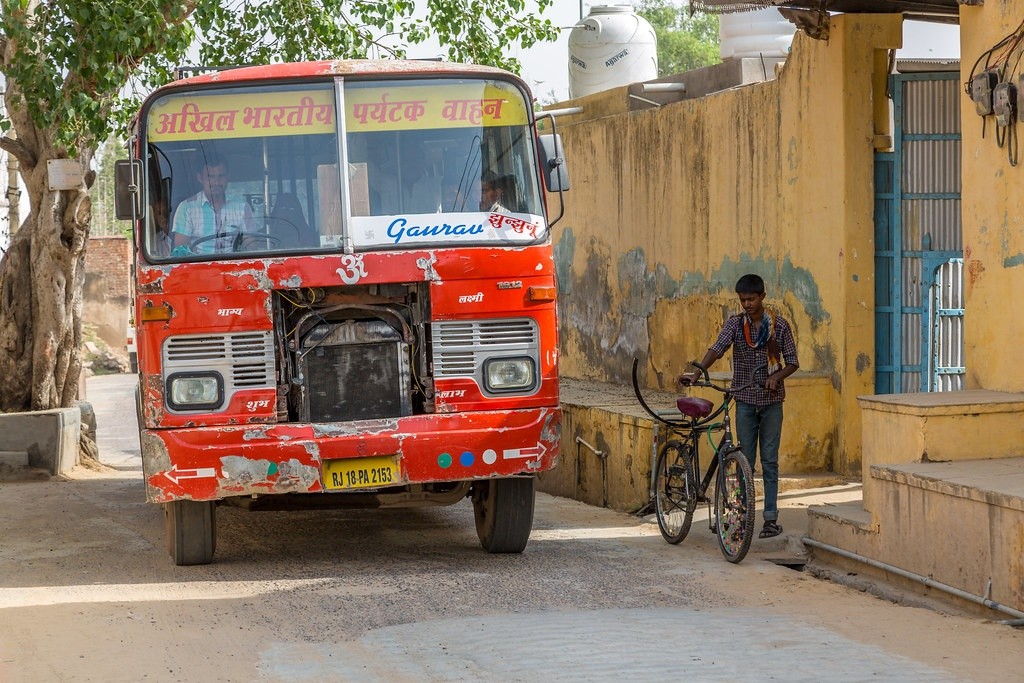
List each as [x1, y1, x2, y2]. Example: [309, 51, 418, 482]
[649, 358, 771, 564]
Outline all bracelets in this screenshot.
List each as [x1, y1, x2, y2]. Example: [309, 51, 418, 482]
[697, 368, 703, 379]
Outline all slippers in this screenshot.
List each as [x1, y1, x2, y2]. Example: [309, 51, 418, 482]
[758, 524, 782, 537]
[731, 528, 744, 539]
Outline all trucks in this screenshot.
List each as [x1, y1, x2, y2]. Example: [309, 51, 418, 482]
[113, 58, 571, 568]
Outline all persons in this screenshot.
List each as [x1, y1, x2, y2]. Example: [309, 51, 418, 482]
[257, 194, 318, 249]
[441, 169, 511, 213]
[151, 197, 176, 255]
[676, 274, 799, 538]
[172, 152, 258, 256]
[370, 146, 443, 213]
